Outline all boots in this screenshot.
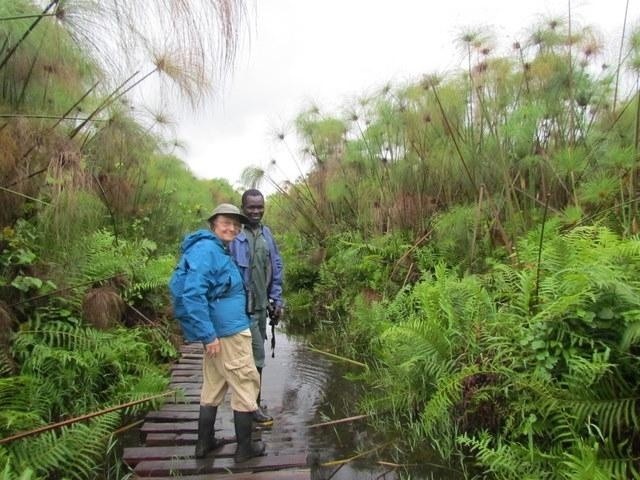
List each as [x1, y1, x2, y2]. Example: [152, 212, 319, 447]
[255, 366, 267, 409]
[194, 404, 226, 458]
[233, 409, 266, 464]
[251, 407, 275, 428]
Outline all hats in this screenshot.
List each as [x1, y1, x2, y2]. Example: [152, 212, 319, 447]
[206, 202, 252, 225]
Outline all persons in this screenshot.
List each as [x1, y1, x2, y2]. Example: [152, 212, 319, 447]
[225, 189, 283, 428]
[168, 202, 266, 463]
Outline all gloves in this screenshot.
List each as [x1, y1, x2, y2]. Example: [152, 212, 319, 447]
[270, 302, 281, 323]
